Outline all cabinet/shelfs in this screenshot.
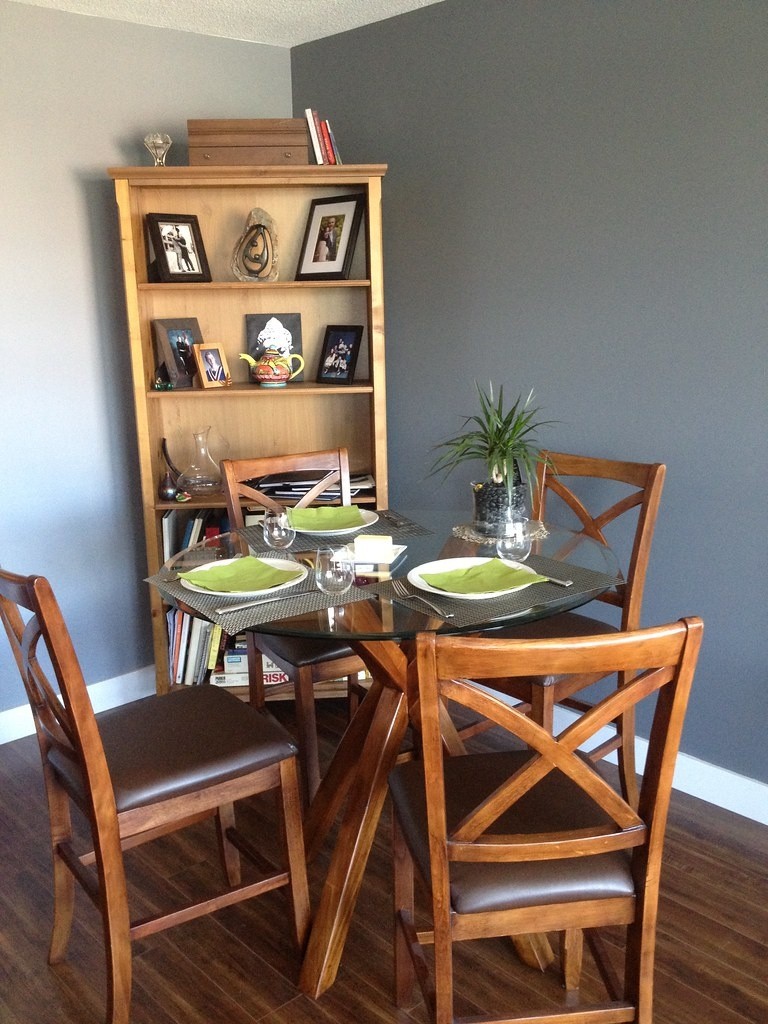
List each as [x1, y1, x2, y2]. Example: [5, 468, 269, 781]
[105, 161, 391, 698]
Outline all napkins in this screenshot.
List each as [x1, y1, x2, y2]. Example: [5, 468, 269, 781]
[177, 554, 302, 595]
[419, 559, 550, 599]
[285, 506, 367, 533]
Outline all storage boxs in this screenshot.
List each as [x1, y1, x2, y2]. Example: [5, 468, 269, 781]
[187, 115, 309, 165]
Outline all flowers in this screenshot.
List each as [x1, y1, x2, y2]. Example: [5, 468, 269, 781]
[435, 376, 570, 486]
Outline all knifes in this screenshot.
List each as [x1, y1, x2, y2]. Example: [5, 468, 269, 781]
[215, 590, 319, 614]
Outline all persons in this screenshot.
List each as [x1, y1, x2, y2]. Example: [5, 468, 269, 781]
[177, 334, 194, 376]
[205, 351, 225, 381]
[318, 218, 337, 261]
[163, 228, 194, 272]
[323, 339, 352, 375]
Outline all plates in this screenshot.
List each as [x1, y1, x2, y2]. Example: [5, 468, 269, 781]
[407, 557, 537, 599]
[330, 542, 408, 565]
[180, 557, 308, 596]
[277, 508, 379, 536]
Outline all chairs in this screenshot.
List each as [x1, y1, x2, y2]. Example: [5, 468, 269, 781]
[386, 613, 708, 1024]
[1, 566, 317, 1023]
[436, 452, 666, 819]
[218, 447, 400, 825]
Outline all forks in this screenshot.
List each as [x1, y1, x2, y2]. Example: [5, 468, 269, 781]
[391, 580, 455, 618]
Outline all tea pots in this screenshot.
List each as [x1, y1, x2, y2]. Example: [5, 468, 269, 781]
[238, 345, 305, 387]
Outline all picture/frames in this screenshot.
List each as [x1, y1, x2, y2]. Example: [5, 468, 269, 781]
[150, 315, 204, 388]
[293, 193, 365, 279]
[194, 342, 231, 387]
[149, 208, 212, 280]
[317, 321, 365, 385]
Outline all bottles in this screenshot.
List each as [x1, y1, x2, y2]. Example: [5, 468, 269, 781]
[176, 425, 222, 494]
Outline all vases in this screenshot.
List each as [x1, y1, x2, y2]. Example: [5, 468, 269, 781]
[472, 483, 529, 538]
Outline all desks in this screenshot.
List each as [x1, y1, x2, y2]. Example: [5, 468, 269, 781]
[156, 509, 624, 1008]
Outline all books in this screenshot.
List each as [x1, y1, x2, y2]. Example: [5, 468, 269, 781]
[166, 606, 367, 687]
[305, 108, 342, 165]
[161, 509, 234, 571]
[239, 471, 375, 501]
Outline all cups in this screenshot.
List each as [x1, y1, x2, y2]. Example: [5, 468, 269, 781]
[315, 544, 354, 596]
[262, 507, 296, 550]
[496, 519, 532, 562]
[317, 593, 355, 635]
[143, 132, 172, 167]
